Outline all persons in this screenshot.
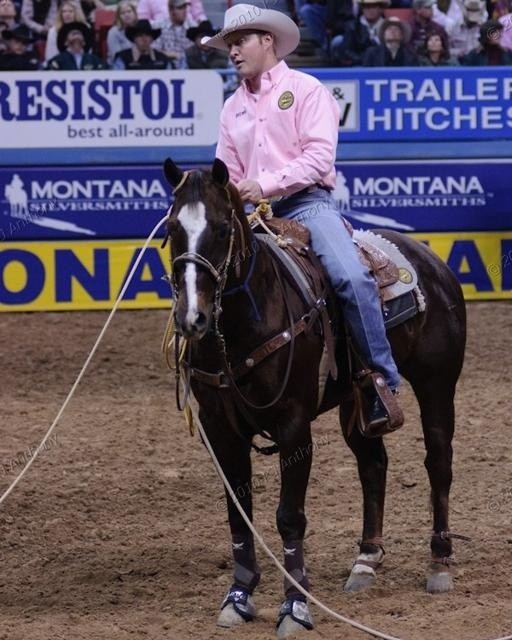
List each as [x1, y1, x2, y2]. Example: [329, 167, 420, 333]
[0, 0, 239, 99]
[294, 0, 512, 67]
[201, 4, 400, 434]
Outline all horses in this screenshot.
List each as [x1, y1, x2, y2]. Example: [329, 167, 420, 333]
[163, 158, 466, 640]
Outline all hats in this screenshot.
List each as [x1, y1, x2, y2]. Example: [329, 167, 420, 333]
[359, 0, 389, 8]
[186, 17, 222, 42]
[199, 2, 301, 62]
[478, 20, 504, 41]
[462, 0, 484, 13]
[378, 17, 412, 47]
[123, 18, 163, 43]
[168, 0, 192, 9]
[1, 23, 40, 45]
[57, 21, 91, 55]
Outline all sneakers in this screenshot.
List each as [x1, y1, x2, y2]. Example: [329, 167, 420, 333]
[365, 390, 399, 425]
